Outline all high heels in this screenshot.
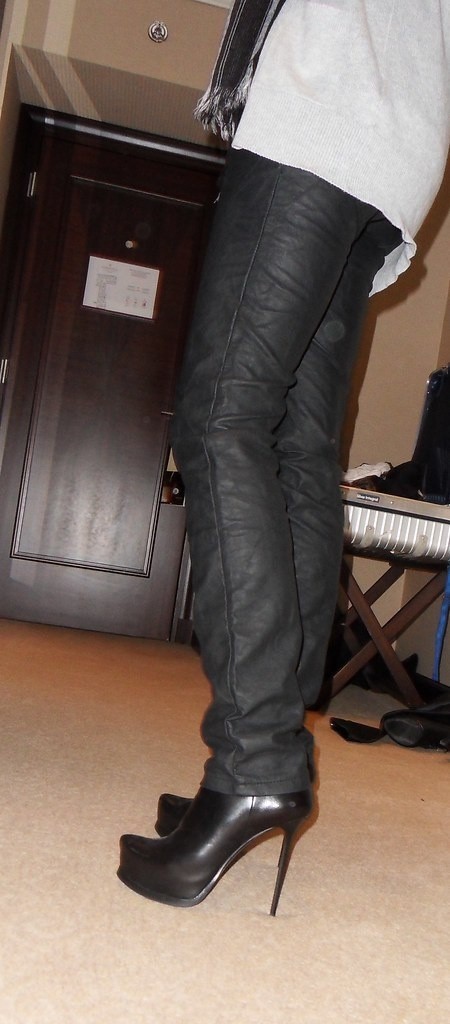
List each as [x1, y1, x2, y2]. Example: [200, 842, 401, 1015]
[117, 785, 312, 918]
[153, 757, 309, 836]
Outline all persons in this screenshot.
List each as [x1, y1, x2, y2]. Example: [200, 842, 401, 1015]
[115, 0, 450, 913]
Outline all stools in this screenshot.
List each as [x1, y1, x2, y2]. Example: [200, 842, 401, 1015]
[331, 541, 450, 710]
